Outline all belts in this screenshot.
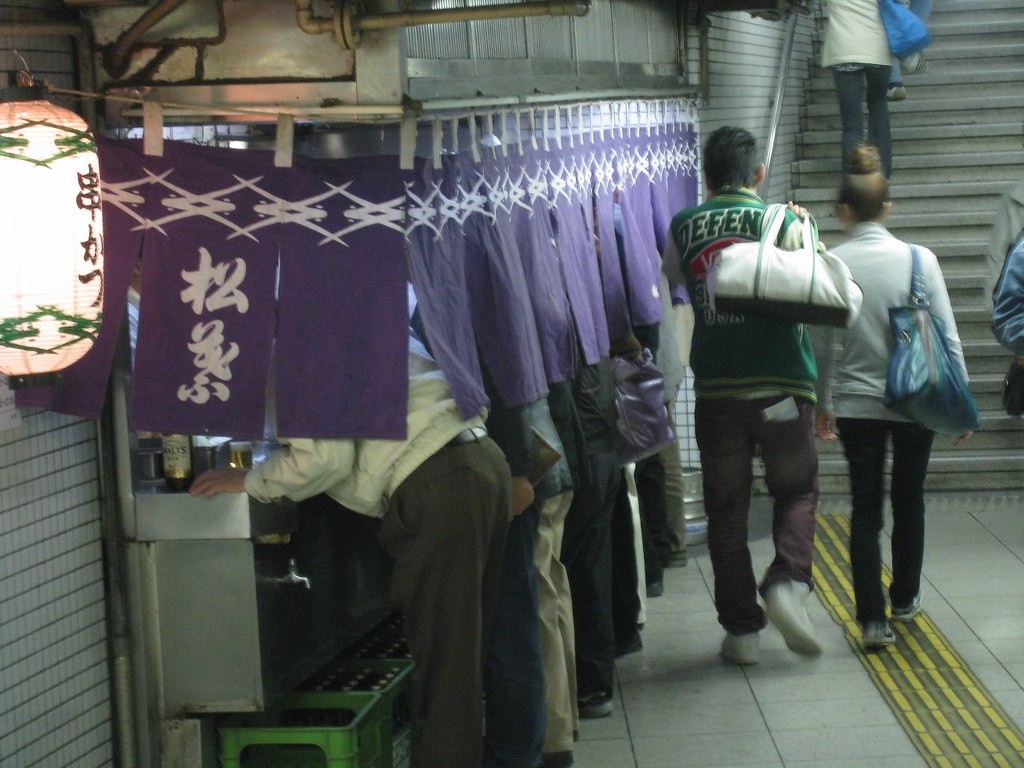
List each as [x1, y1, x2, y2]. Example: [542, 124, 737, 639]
[445, 427, 489, 448]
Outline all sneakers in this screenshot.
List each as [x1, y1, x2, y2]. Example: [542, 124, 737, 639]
[891, 586, 924, 622]
[860, 620, 896, 647]
[765, 583, 825, 657]
[721, 629, 762, 663]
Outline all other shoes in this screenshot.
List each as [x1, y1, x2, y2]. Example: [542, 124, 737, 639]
[610, 632, 643, 658]
[661, 556, 688, 568]
[576, 691, 613, 717]
[536, 751, 573, 768]
[644, 579, 662, 597]
[885, 81, 906, 101]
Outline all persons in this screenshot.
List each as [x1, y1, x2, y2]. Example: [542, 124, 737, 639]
[188, 300, 581, 768]
[813, 139, 973, 648]
[822, 0, 934, 187]
[660, 125, 823, 668]
[591, 189, 687, 596]
[570, 334, 642, 718]
[984, 177, 1024, 369]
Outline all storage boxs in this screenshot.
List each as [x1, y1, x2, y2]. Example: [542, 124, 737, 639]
[217, 693, 387, 768]
[304, 661, 418, 768]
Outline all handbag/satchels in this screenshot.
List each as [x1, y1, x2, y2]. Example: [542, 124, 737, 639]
[878, 0, 932, 59]
[881, 244, 981, 438]
[608, 347, 674, 465]
[1001, 363, 1024, 415]
[526, 424, 560, 488]
[707, 201, 862, 329]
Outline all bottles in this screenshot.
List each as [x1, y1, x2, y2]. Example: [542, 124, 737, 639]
[161, 432, 195, 491]
[260, 608, 412, 746]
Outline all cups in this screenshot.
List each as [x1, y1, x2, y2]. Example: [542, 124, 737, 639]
[231, 442, 252, 472]
